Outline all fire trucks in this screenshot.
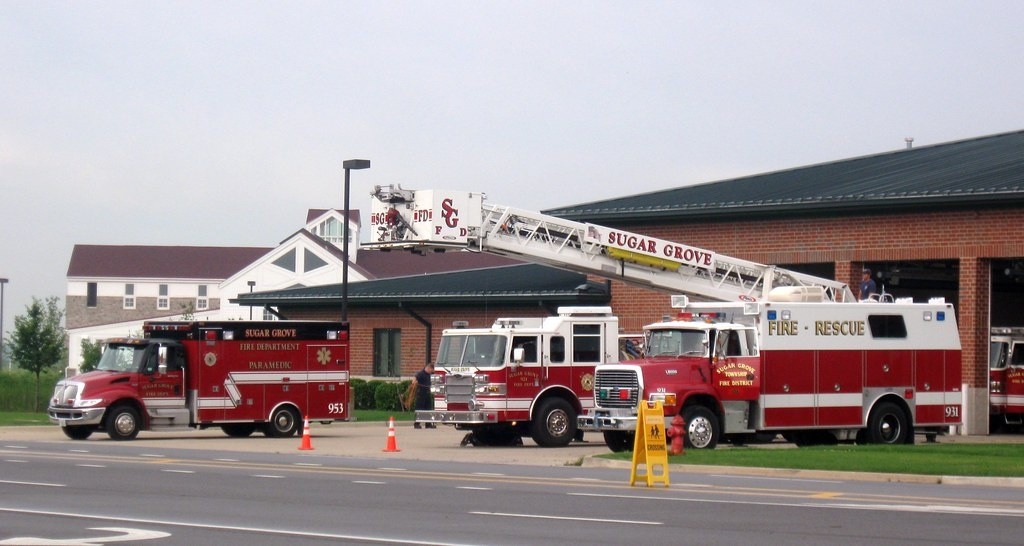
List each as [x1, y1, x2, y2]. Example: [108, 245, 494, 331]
[414, 306, 674, 449]
[989, 324, 1024, 431]
[360, 182, 964, 453]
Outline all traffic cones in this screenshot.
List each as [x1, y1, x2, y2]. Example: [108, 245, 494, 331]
[296, 415, 315, 450]
[381, 416, 404, 452]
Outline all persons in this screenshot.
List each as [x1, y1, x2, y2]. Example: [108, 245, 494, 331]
[412, 363, 436, 429]
[859, 269, 876, 299]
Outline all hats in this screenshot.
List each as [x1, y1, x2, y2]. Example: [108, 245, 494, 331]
[862, 269, 871, 273]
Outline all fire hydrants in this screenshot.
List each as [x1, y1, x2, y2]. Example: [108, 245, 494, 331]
[666, 413, 687, 455]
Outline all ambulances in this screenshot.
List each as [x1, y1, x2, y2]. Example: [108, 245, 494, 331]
[47, 317, 353, 442]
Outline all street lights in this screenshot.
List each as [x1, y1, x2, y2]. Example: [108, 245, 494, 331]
[0, 278, 10, 367]
[247, 279, 257, 320]
[339, 158, 372, 321]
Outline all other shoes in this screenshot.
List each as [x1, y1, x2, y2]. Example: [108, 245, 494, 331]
[414, 426, 421, 429]
[426, 425, 436, 428]
